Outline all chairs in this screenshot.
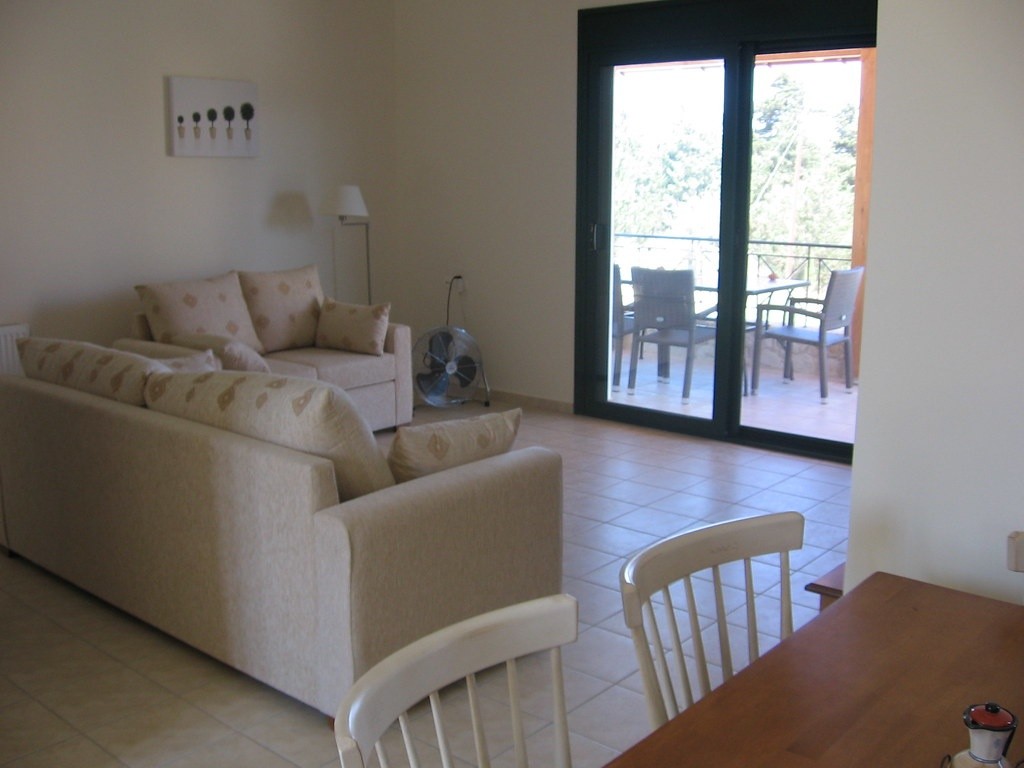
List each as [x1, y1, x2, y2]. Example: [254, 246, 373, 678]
[627, 266, 718, 405]
[613, 265, 664, 392]
[750, 266, 865, 404]
[334, 594, 580, 768]
[619, 512, 805, 732]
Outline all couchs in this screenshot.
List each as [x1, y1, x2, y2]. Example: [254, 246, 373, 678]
[113, 262, 412, 432]
[0, 336, 564, 732]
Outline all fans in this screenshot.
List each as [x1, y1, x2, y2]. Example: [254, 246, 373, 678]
[411, 326, 491, 416]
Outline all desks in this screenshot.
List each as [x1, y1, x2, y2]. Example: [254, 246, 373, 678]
[599, 572, 1024, 768]
[621, 275, 811, 395]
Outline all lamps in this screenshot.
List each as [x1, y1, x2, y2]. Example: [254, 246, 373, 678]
[319, 185, 372, 308]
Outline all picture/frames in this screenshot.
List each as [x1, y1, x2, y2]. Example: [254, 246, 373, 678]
[167, 74, 262, 158]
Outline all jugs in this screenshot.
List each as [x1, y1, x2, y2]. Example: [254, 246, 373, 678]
[939, 702, 1024, 768]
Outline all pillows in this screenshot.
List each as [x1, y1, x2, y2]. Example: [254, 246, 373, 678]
[172, 333, 270, 374]
[317, 296, 391, 357]
[160, 348, 216, 374]
[387, 407, 523, 484]
